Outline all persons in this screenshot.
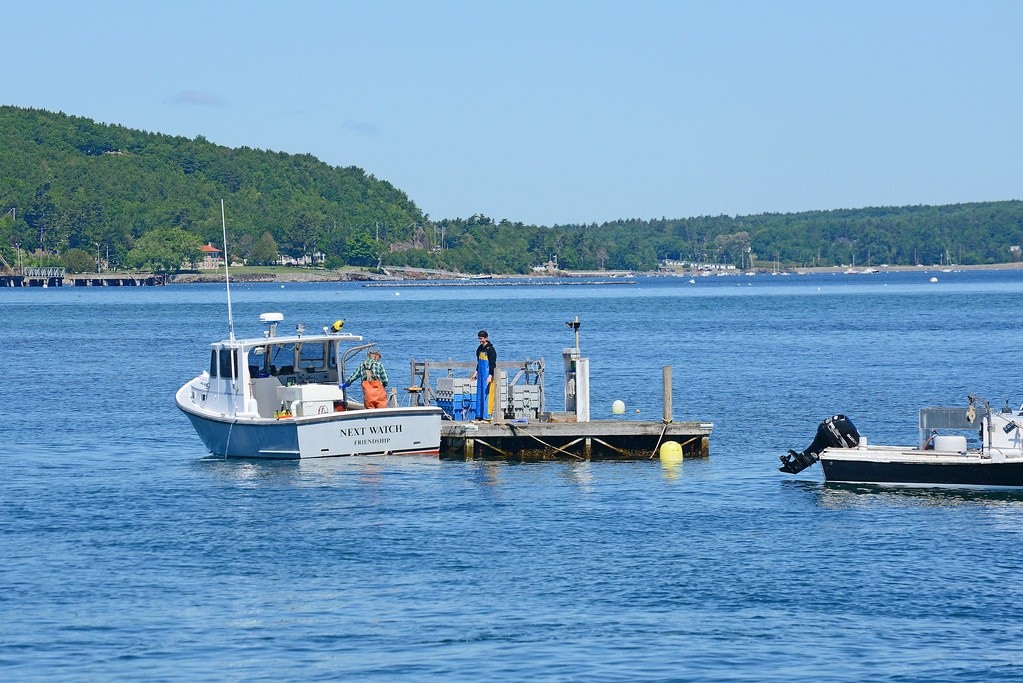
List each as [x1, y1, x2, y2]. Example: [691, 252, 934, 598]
[339, 345, 388, 409]
[469, 331, 497, 421]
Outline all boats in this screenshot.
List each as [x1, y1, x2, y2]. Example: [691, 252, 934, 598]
[779, 395, 1023, 494]
[174, 199, 443, 460]
[860, 267, 879, 275]
[844, 268, 859, 274]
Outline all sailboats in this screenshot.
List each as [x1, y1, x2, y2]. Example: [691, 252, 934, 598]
[745, 253, 757, 276]
[772, 251, 790, 276]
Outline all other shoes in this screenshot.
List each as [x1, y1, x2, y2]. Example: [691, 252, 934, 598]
[480, 419, 492, 424]
[471, 418, 483, 424]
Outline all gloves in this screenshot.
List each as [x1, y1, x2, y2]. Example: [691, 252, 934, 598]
[339, 381, 349, 389]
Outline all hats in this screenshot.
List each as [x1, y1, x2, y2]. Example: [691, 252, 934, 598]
[367, 347, 382, 358]
